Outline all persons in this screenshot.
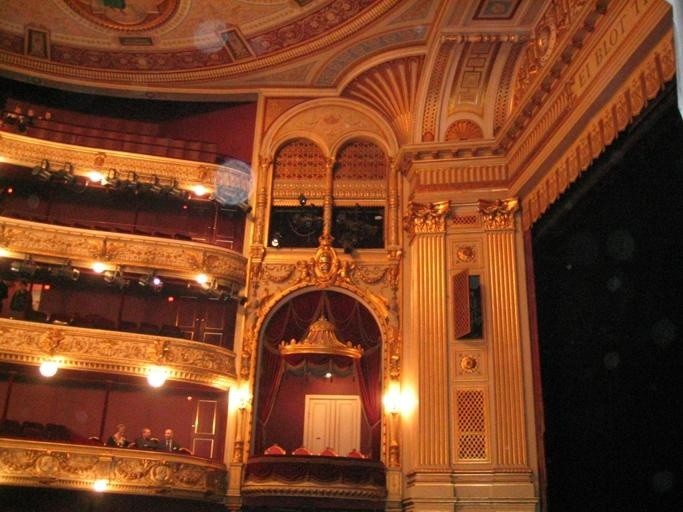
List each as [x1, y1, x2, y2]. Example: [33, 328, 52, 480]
[135, 427, 160, 450]
[106, 419, 137, 449]
[38, 109, 55, 120]
[9, 277, 33, 312]
[158, 428, 180, 451]
[0, 270, 14, 316]
[27, 107, 37, 120]
[0, 98, 27, 128]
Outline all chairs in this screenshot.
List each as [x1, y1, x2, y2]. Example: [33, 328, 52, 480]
[0, 212, 191, 243]
[1, 307, 185, 340]
[262, 442, 368, 459]
[0, 418, 193, 456]
